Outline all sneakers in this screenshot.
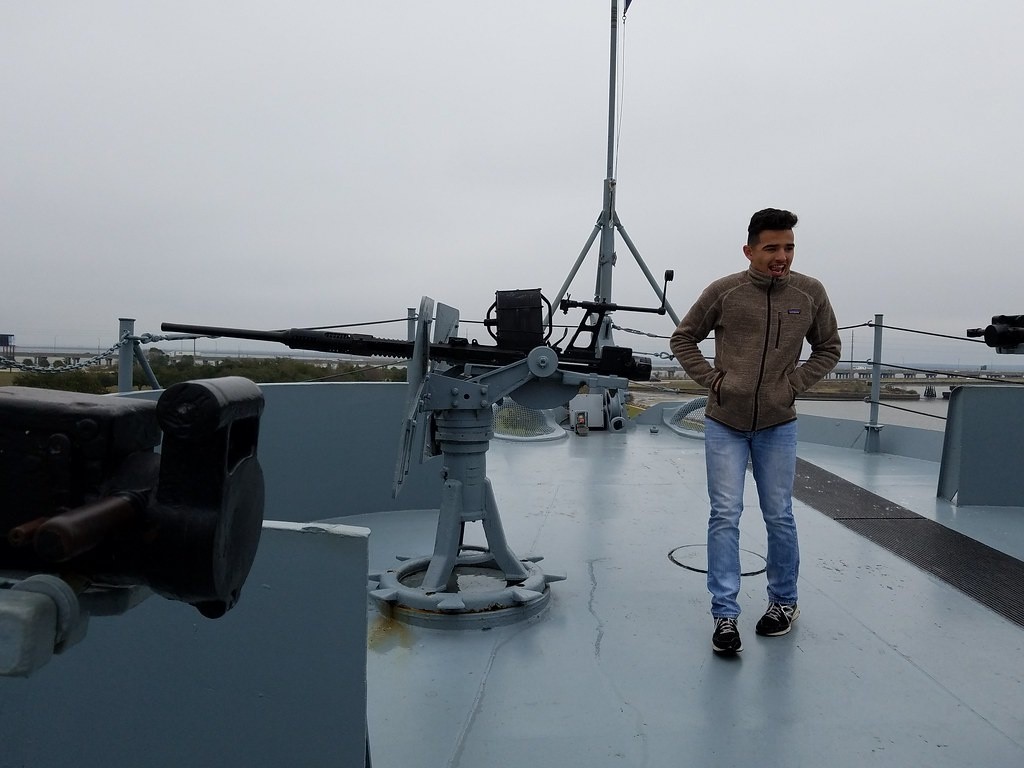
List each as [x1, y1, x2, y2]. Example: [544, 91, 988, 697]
[712, 615, 744, 656]
[755, 598, 800, 638]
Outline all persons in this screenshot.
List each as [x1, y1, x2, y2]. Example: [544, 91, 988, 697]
[669, 208, 842, 652]
